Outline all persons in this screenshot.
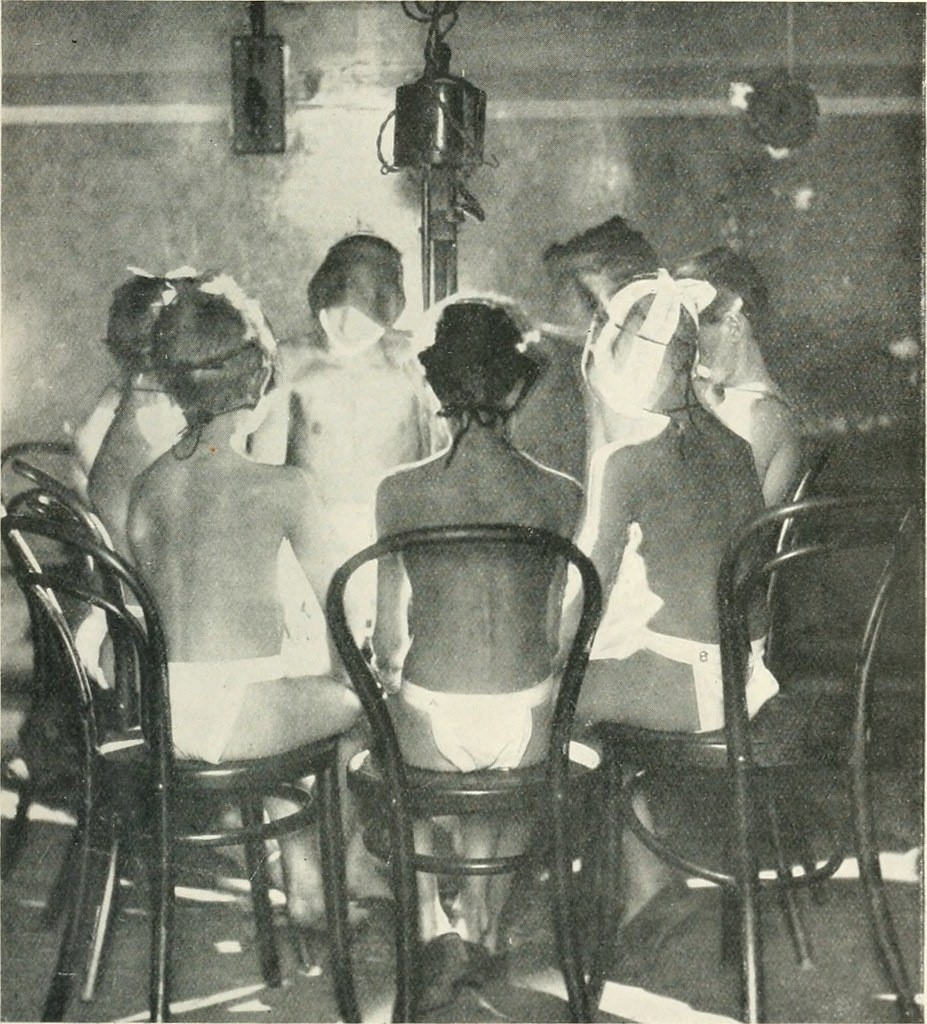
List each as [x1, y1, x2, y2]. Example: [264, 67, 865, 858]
[120, 272, 393, 929]
[74, 272, 282, 894]
[352, 294, 592, 1013]
[65, 215, 800, 687]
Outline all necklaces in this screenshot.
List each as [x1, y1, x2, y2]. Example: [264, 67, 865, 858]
[555, 274, 779, 960]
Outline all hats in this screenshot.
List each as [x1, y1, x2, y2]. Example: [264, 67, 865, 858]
[307, 236, 405, 331]
[150, 274, 274, 416]
[104, 266, 195, 371]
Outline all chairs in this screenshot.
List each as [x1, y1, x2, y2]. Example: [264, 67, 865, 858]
[0, 439, 364, 1024]
[585, 437, 925, 1024]
[323, 524, 604, 1024]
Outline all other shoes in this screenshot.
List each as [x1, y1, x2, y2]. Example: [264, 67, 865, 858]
[614, 880, 701, 978]
[463, 941, 490, 986]
[416, 932, 469, 1010]
